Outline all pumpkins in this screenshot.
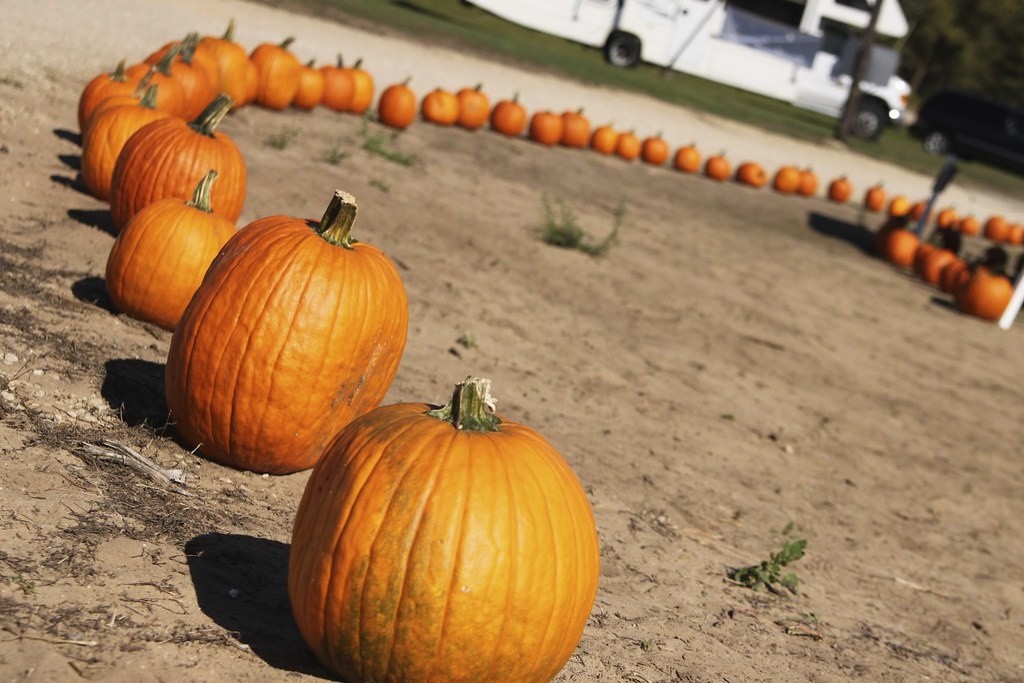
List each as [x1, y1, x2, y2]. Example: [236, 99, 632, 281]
[78, 20, 524, 473]
[529, 108, 1024, 320]
[287, 372, 599, 683]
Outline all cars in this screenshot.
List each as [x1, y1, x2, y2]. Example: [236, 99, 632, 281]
[905, 87, 1023, 180]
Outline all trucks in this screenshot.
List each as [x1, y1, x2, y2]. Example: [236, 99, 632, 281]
[467, 1, 910, 142]
